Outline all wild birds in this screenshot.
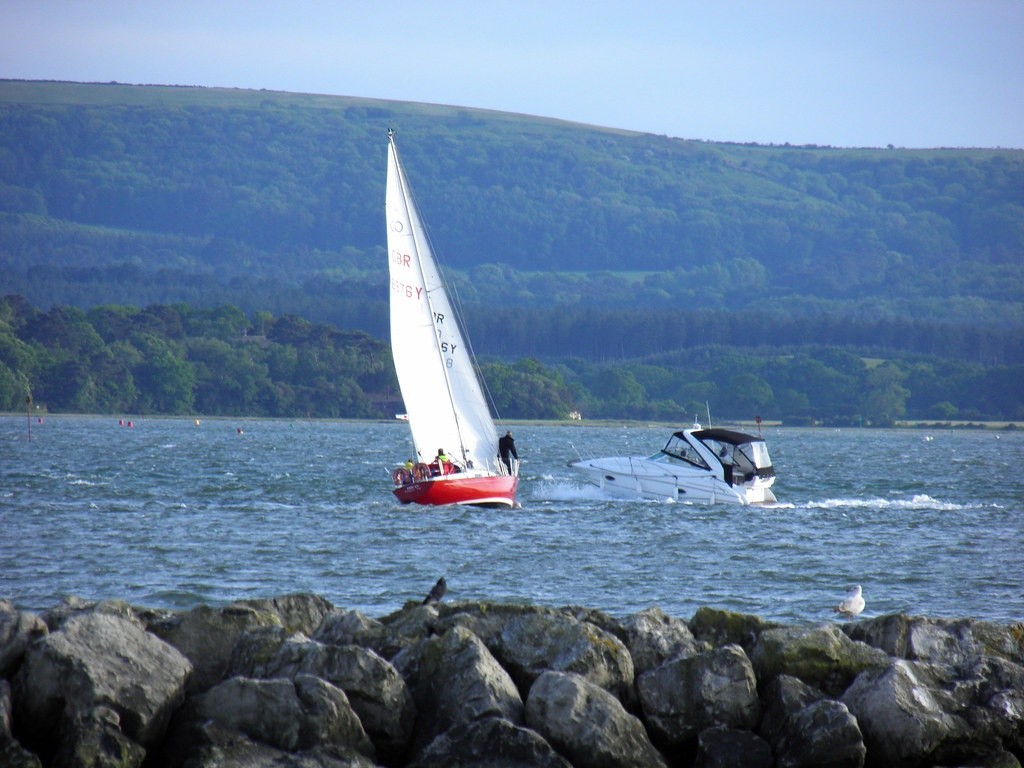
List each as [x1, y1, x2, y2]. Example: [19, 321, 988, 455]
[422, 576, 447, 607]
[834, 583, 866, 621]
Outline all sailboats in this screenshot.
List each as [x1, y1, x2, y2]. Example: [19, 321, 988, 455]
[384, 128, 521, 508]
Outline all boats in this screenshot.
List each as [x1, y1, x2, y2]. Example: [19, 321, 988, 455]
[567, 400, 778, 505]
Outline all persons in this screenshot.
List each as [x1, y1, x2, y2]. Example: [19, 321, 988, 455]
[497, 431, 520, 475]
[405, 459, 415, 472]
[434, 448, 448, 464]
